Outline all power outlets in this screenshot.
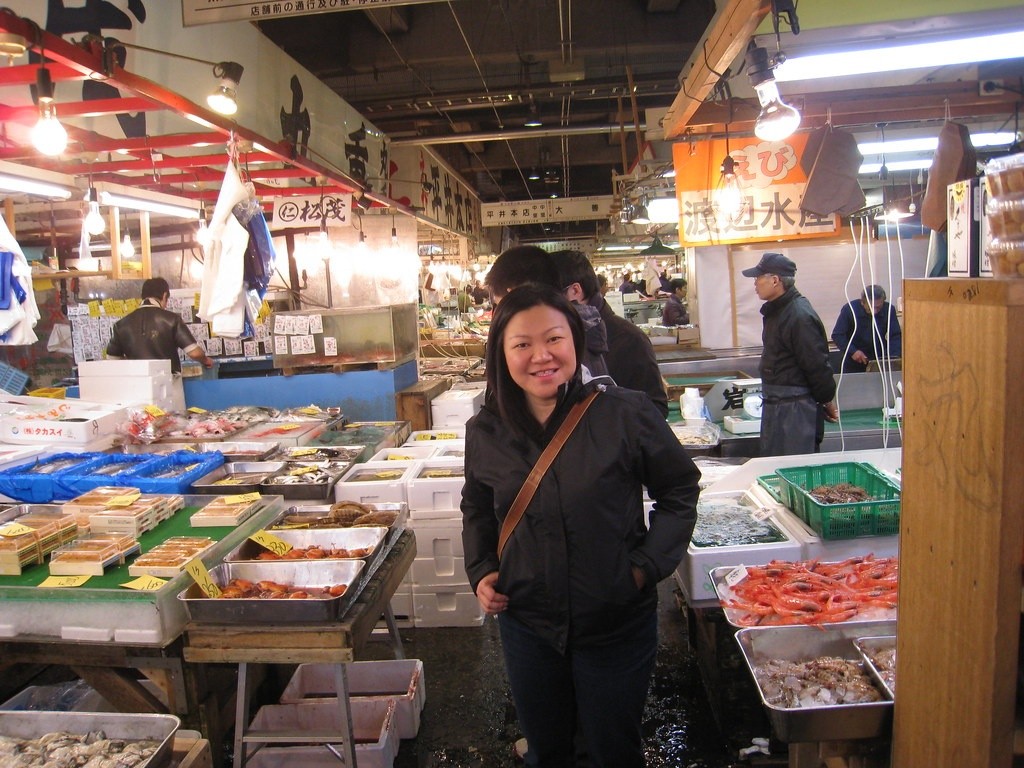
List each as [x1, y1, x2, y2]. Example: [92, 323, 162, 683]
[978, 79, 1005, 96]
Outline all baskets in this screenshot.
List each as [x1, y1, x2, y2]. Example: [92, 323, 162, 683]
[756, 462, 901, 541]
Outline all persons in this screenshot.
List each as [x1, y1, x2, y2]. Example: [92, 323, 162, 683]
[659, 269, 675, 292]
[619, 274, 638, 323]
[831, 285, 902, 374]
[105, 277, 212, 376]
[458, 280, 489, 317]
[742, 252, 839, 457]
[460, 285, 702, 768]
[597, 274, 608, 298]
[663, 278, 690, 327]
[548, 250, 669, 420]
[485, 246, 610, 377]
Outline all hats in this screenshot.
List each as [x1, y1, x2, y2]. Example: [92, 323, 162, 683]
[742, 252, 796, 277]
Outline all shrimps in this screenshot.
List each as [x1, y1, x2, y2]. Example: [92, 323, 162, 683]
[717, 546, 917, 720]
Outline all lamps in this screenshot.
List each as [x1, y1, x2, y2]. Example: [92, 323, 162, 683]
[365, 178, 432, 193]
[97, 36, 245, 114]
[294, 142, 374, 210]
[859, 159, 933, 174]
[0, 172, 74, 200]
[873, 175, 913, 222]
[101, 191, 199, 219]
[857, 131, 1021, 155]
[641, 231, 676, 256]
[773, 23, 1024, 82]
[630, 187, 651, 225]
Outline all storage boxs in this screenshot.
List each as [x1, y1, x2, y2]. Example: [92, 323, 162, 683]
[777, 508, 899, 563]
[708, 559, 899, 741]
[623, 293, 639, 302]
[611, 306, 624, 317]
[281, 659, 426, 739]
[270, 302, 417, 370]
[246, 698, 399, 768]
[405, 518, 465, 557]
[644, 490, 801, 601]
[374, 563, 413, 627]
[431, 389, 484, 425]
[334, 460, 415, 504]
[412, 557, 474, 592]
[672, 328, 699, 345]
[407, 458, 466, 519]
[774, 461, 901, 540]
[78, 359, 170, 407]
[413, 592, 485, 627]
[604, 291, 623, 306]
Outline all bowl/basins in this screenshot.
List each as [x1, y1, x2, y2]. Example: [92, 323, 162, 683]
[985, 153, 1024, 279]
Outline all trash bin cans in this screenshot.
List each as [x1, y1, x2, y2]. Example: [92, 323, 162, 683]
[732, 378, 764, 422]
